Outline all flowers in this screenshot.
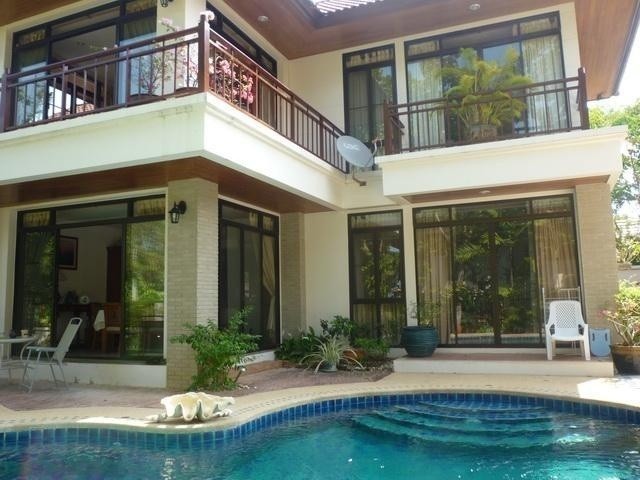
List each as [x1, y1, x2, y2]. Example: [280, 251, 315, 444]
[599, 295, 639, 346]
[105, 18, 253, 107]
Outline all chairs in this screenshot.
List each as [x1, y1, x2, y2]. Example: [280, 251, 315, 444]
[91, 303, 121, 353]
[21, 317, 83, 396]
[544, 300, 591, 360]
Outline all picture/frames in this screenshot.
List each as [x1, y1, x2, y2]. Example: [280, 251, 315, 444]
[59, 235, 78, 270]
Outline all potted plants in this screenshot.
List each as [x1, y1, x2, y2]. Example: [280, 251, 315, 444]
[430, 47, 534, 138]
[402, 300, 442, 356]
[275, 316, 389, 374]
[168, 306, 264, 390]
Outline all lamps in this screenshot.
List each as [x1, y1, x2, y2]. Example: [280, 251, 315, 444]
[169, 200, 186, 224]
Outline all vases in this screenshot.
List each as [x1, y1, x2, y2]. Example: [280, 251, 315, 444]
[609, 343, 640, 375]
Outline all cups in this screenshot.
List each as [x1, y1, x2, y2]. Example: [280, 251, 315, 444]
[8, 329, 17, 339]
[1, 330, 9, 339]
[21, 329, 30, 339]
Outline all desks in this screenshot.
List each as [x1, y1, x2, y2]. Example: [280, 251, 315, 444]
[2, 335, 39, 389]
[58, 303, 89, 348]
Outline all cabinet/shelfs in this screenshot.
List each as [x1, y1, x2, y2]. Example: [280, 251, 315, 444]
[107, 247, 121, 301]
